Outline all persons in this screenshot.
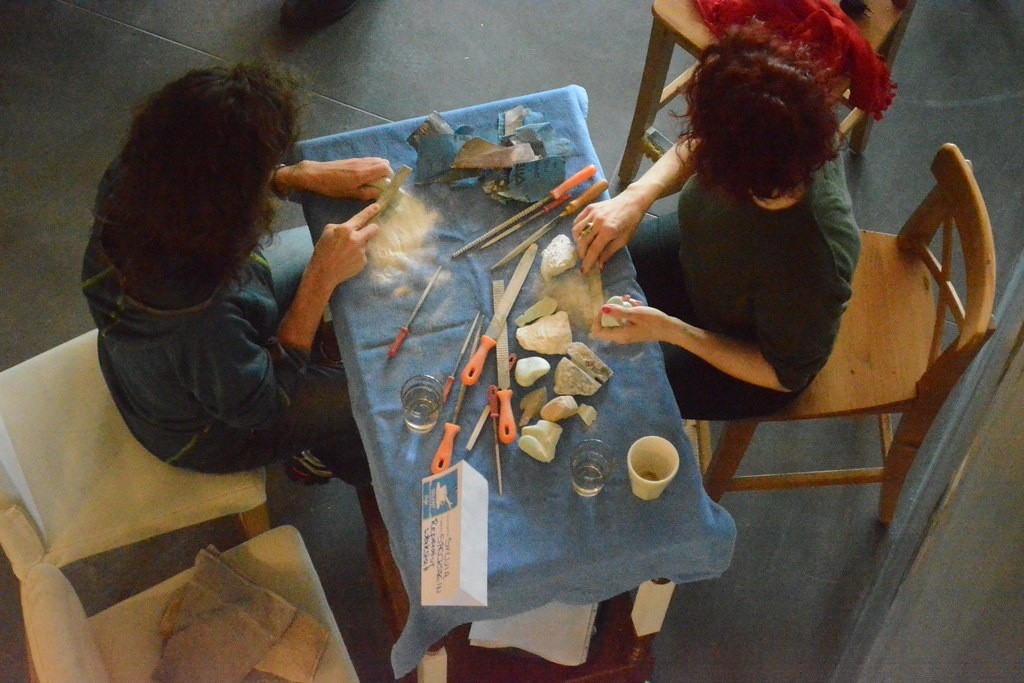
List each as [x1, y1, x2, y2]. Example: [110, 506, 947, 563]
[570, 38, 860, 422]
[82, 63, 395, 485]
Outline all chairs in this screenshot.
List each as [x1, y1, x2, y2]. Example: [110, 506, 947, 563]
[685, 141, 998, 525]
[618, 0, 916, 181]
[0, 329, 275, 582]
[20, 525, 358, 683]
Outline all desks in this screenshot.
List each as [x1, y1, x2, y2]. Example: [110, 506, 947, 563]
[284, 84, 736, 683]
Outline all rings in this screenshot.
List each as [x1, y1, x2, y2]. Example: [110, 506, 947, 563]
[581, 222, 594, 236]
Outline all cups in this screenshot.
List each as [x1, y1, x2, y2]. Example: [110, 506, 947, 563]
[570, 439, 616, 497]
[627, 435, 680, 500]
[401, 375, 445, 435]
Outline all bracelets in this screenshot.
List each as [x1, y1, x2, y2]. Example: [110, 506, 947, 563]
[269, 164, 288, 201]
